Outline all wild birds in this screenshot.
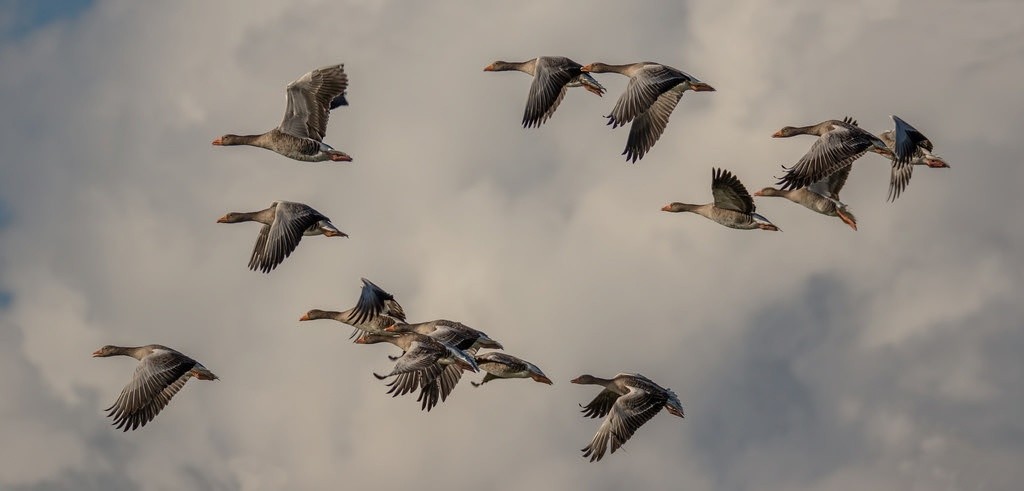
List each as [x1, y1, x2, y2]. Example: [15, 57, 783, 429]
[92, 344, 222, 433]
[211, 64, 353, 162]
[772, 116, 899, 192]
[660, 167, 784, 232]
[569, 373, 685, 463]
[482, 56, 607, 128]
[754, 163, 857, 232]
[867, 116, 950, 203]
[216, 201, 349, 275]
[298, 278, 553, 412]
[580, 61, 717, 164]
